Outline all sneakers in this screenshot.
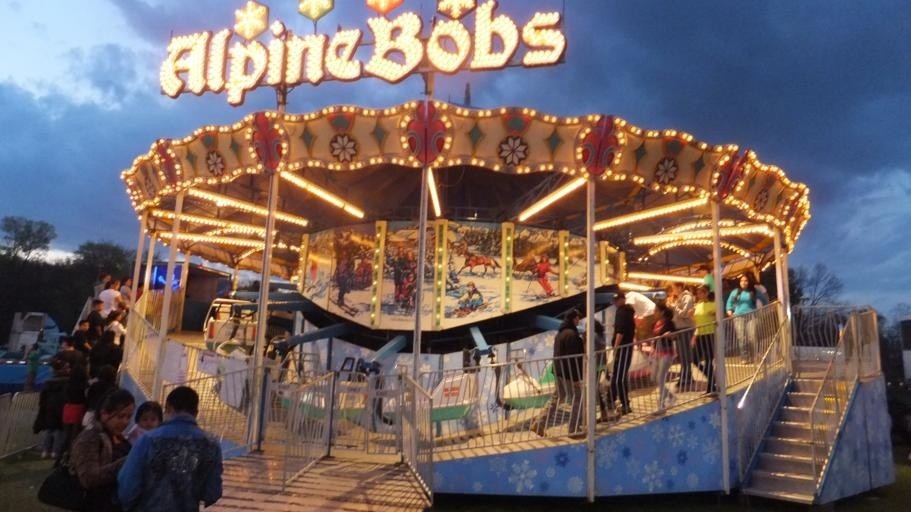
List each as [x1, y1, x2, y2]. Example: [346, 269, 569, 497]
[530, 424, 547, 437]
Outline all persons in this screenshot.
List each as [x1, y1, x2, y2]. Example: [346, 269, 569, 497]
[64, 388, 133, 510]
[604, 292, 635, 421]
[125, 401, 163, 447]
[118, 386, 222, 511]
[531, 308, 585, 439]
[41, 272, 146, 461]
[649, 264, 769, 417]
[25, 342, 39, 391]
[583, 319, 606, 424]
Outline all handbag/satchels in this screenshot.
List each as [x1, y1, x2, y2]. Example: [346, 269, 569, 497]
[37, 466, 88, 512]
